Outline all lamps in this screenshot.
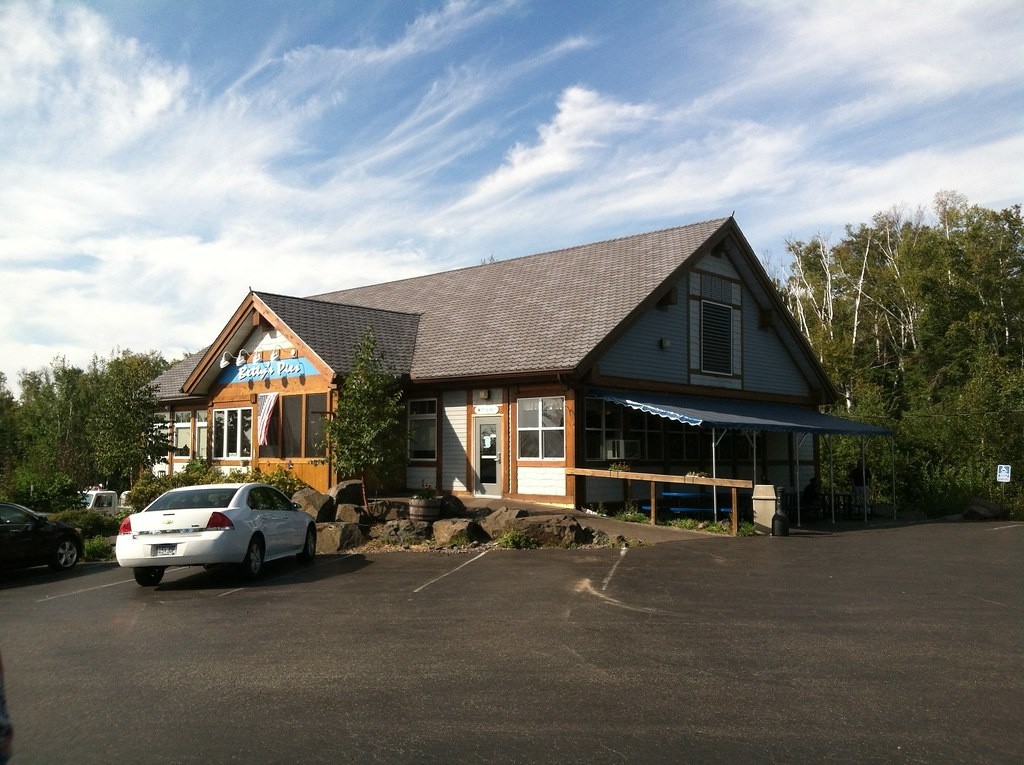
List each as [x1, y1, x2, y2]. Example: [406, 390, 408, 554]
[219, 344, 297, 367]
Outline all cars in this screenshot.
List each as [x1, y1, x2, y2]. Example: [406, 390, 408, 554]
[0, 500, 85, 577]
[115, 482, 318, 588]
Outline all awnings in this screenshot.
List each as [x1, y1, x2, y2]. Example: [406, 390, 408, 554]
[588, 391, 895, 438]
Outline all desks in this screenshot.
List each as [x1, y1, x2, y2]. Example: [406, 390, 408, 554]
[660, 491, 713, 508]
[794, 492, 854, 521]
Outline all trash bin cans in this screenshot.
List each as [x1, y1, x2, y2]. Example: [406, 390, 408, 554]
[752, 485, 777, 537]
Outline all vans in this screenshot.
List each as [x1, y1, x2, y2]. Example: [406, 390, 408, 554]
[76, 490, 134, 510]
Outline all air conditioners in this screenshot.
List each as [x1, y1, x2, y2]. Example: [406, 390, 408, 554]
[606, 440, 641, 459]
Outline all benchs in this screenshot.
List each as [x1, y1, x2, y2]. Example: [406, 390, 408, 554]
[842, 504, 876, 520]
[783, 505, 822, 524]
[641, 506, 732, 513]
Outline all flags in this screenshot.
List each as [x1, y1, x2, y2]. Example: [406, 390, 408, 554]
[246, 392, 279, 448]
